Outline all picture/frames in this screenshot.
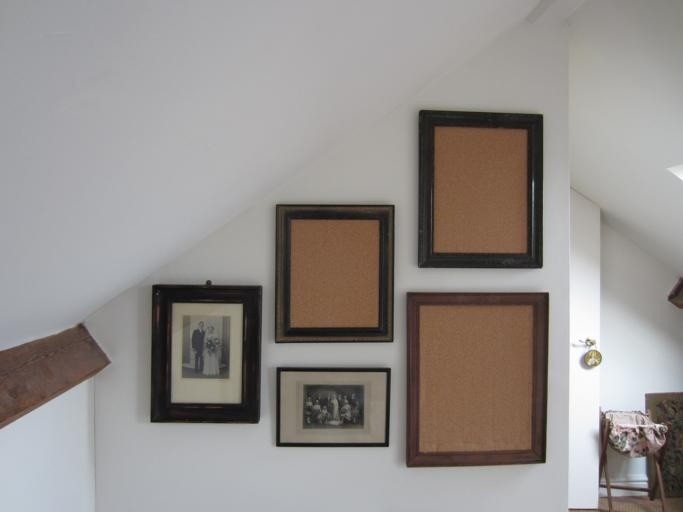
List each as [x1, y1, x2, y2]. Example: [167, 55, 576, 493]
[275, 367, 391, 447]
[149, 283, 262, 424]
[418, 108, 543, 270]
[406, 291, 538, 451]
[275, 204, 393, 344]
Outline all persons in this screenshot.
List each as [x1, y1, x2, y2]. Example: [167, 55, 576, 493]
[304, 390, 361, 426]
[190, 320, 204, 374]
[201, 322, 223, 376]
